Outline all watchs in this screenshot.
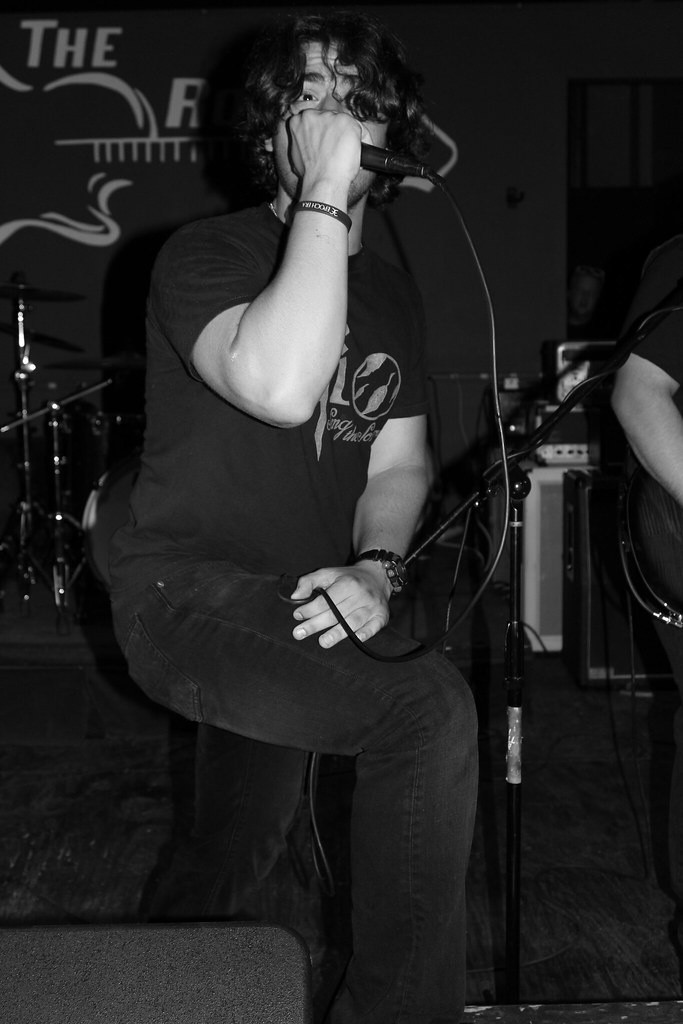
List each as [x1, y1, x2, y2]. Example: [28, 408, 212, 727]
[352, 549, 408, 599]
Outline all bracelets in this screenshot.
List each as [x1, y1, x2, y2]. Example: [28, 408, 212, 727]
[289, 201, 352, 234]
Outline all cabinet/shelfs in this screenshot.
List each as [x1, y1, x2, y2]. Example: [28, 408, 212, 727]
[565, 76, 683, 343]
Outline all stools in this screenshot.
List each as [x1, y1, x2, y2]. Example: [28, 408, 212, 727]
[64, 584, 363, 1024]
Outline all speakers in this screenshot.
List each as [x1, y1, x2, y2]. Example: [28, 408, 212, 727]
[495, 338, 671, 689]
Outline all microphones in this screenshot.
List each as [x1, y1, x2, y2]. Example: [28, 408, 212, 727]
[360, 141, 432, 180]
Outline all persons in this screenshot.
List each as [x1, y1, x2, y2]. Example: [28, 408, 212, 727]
[566, 247, 608, 317]
[106, 5, 483, 1023]
[609, 233, 683, 950]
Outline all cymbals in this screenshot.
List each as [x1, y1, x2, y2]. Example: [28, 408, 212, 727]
[43, 357, 120, 368]
[1, 324, 86, 352]
[0, 282, 84, 303]
